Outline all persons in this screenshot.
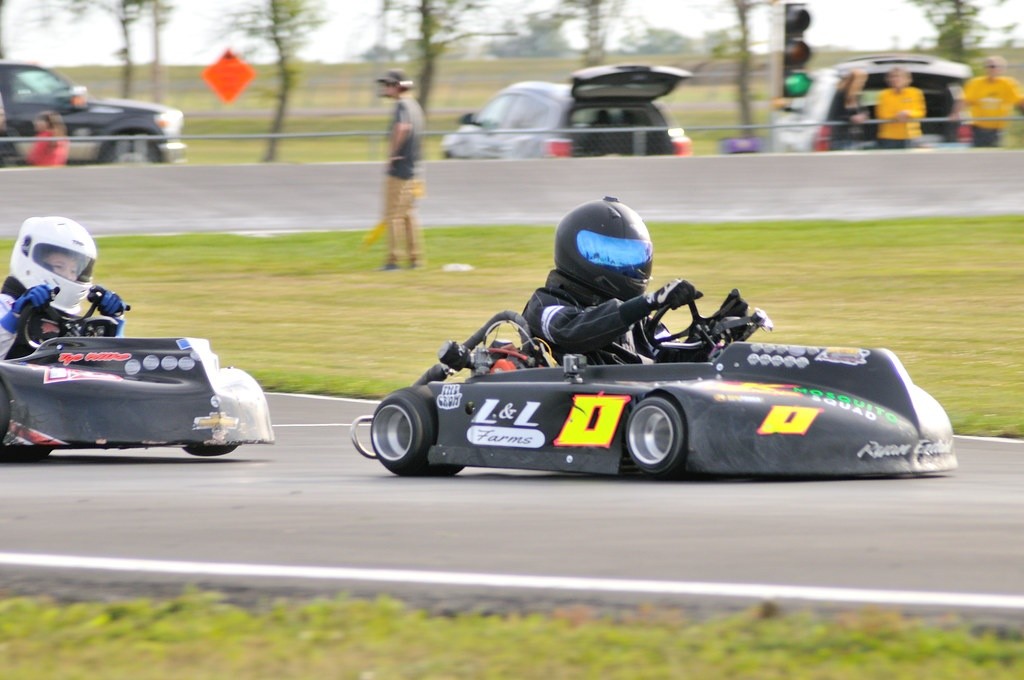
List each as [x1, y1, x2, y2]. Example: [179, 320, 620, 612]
[519, 196, 749, 366]
[0, 105, 69, 168]
[961, 57, 1024, 148]
[0, 215, 126, 363]
[875, 67, 927, 147]
[828, 67, 869, 147]
[377, 69, 423, 269]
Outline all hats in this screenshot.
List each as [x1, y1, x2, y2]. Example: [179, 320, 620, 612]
[374, 68, 411, 85]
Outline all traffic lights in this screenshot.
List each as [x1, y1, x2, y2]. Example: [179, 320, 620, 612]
[782, 4, 812, 99]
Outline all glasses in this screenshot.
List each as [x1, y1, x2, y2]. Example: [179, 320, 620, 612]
[985, 66, 997, 69]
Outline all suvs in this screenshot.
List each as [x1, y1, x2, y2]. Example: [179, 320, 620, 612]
[775, 56, 977, 150]
[1, 60, 187, 167]
[439, 62, 697, 158]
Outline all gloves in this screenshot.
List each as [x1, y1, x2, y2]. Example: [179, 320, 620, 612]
[0, 284, 55, 334]
[88, 285, 124, 317]
[713, 289, 749, 321]
[650, 276, 703, 310]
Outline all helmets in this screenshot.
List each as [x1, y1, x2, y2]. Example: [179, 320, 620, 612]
[553, 196, 654, 301]
[10, 216, 97, 314]
